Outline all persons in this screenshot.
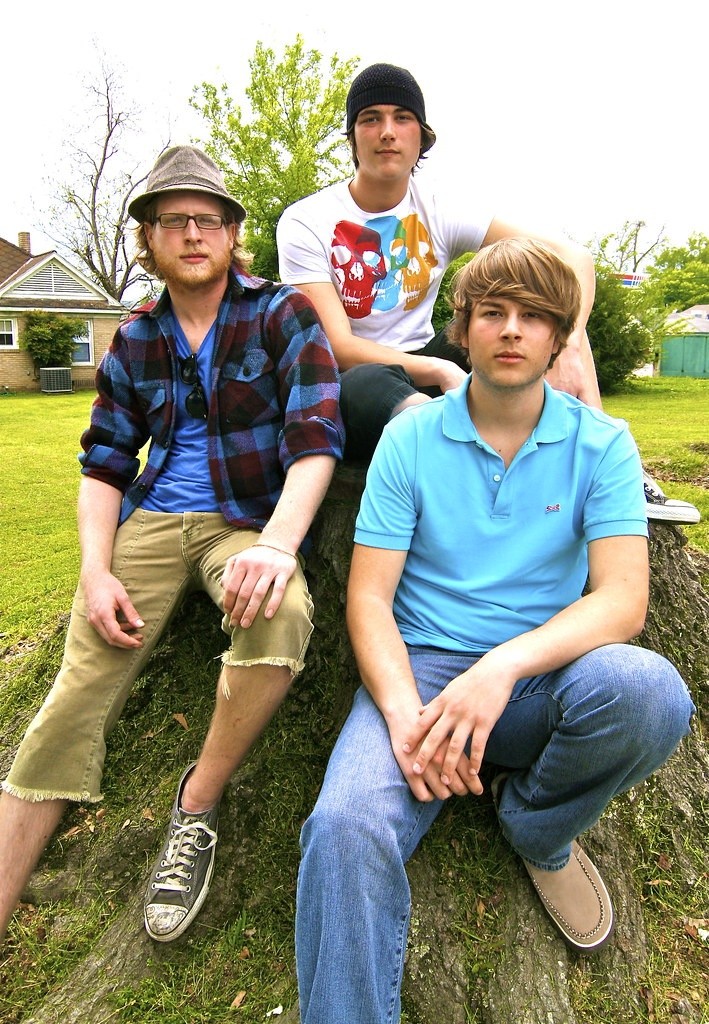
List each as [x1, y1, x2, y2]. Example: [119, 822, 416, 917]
[274, 64, 702, 528]
[294, 237, 696, 1024]
[0, 145, 345, 943]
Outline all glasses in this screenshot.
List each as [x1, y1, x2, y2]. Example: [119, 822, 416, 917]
[152, 212, 229, 230]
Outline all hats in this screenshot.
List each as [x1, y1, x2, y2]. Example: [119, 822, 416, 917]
[128, 145, 247, 224]
[347, 63, 436, 154]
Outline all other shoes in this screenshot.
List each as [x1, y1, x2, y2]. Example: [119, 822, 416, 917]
[492, 773, 612, 947]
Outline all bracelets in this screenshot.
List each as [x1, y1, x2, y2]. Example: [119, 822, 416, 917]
[253, 543, 298, 562]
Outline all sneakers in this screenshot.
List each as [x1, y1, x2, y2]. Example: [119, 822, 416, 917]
[143, 761, 219, 942]
[642, 468, 701, 525]
